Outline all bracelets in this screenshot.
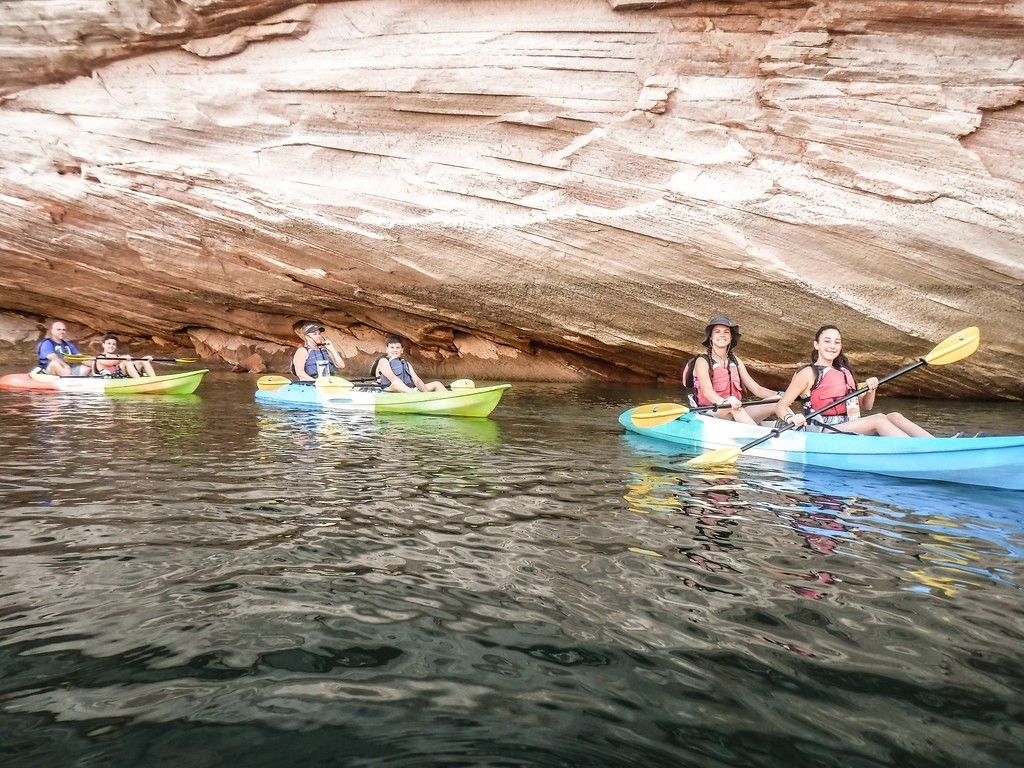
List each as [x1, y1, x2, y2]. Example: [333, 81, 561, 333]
[784, 414, 793, 422]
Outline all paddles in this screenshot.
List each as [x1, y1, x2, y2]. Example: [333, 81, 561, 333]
[256, 375, 379, 392]
[28, 373, 149, 382]
[686, 325, 980, 464]
[631, 380, 867, 429]
[63, 354, 200, 364]
[315, 376, 476, 394]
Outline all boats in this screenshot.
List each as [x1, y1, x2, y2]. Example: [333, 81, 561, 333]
[619, 403, 1024, 493]
[0, 369, 210, 395]
[255, 383, 511, 419]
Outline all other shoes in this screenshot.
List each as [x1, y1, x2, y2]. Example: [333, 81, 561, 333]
[950, 432, 965, 438]
[973, 432, 986, 438]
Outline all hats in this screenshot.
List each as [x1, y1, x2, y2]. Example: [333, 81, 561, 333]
[702, 316, 742, 351]
[304, 326, 325, 336]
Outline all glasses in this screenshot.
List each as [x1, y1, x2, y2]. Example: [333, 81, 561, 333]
[310, 335, 326, 346]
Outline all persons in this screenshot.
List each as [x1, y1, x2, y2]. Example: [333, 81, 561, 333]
[290, 325, 345, 381]
[370, 338, 447, 393]
[92, 335, 155, 379]
[775, 325, 986, 438]
[38, 322, 92, 376]
[682, 316, 794, 426]
